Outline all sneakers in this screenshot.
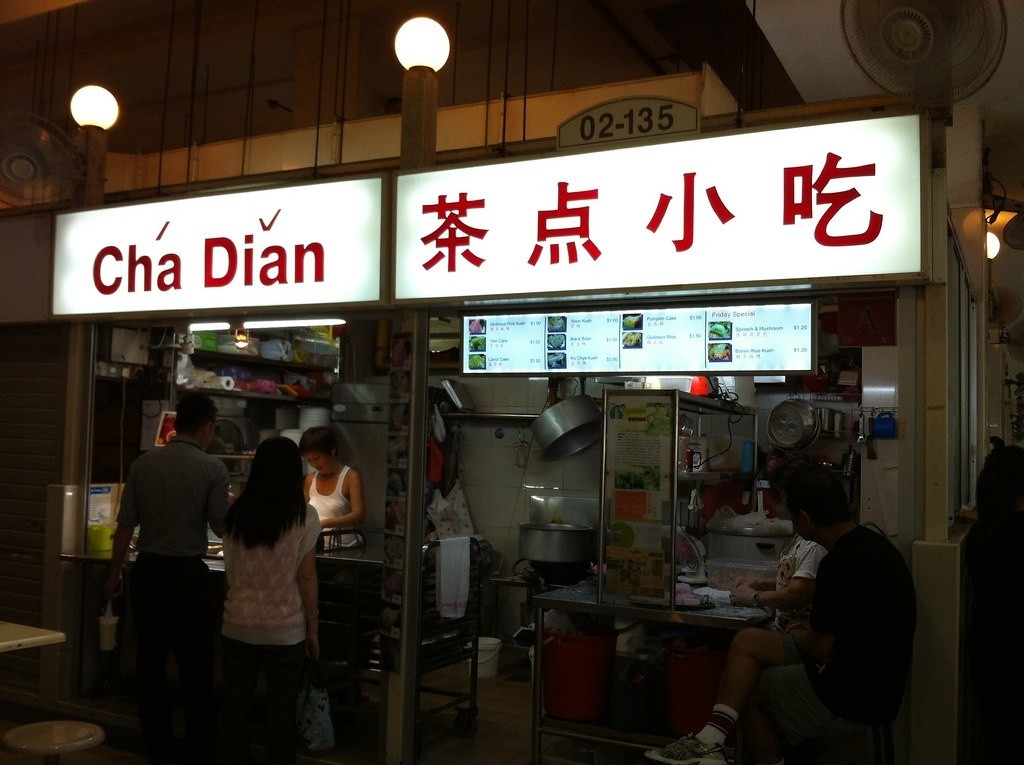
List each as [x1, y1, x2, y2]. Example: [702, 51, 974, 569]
[645, 732, 728, 765]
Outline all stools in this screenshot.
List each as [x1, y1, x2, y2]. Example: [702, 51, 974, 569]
[5, 721, 105, 765]
[841, 712, 897, 765]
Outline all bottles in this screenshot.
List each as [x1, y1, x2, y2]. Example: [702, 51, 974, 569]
[741, 440, 753, 473]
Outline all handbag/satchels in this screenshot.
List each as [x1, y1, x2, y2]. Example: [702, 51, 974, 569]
[295, 653, 336, 750]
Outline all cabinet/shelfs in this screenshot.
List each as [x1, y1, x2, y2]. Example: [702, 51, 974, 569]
[598, 390, 758, 611]
[167, 346, 340, 459]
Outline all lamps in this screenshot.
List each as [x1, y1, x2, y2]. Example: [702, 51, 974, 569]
[235, 328, 248, 350]
[70, 85, 118, 129]
[987, 231, 1000, 259]
[394, 17, 451, 72]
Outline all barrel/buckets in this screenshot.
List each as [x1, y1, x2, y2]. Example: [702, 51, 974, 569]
[466, 637, 501, 678]
[529, 646, 534, 688]
[543, 628, 729, 733]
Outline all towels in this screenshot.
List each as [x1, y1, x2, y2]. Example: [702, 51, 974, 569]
[434, 536, 471, 618]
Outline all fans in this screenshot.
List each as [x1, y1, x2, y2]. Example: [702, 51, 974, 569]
[984, 193, 1024, 250]
[840, 0, 1008, 126]
[0, 110, 87, 213]
[1000, 319, 1024, 363]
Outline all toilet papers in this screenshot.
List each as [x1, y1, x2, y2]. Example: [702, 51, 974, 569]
[206, 376, 235, 391]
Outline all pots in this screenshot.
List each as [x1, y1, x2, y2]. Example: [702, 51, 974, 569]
[707, 529, 794, 569]
[767, 391, 822, 455]
[516, 522, 597, 565]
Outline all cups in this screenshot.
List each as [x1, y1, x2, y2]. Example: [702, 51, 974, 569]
[99, 616, 118, 650]
[869, 413, 895, 437]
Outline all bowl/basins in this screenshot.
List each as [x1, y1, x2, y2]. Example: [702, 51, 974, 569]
[530, 394, 601, 462]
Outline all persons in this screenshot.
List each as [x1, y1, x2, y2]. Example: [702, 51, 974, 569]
[645, 453, 919, 765]
[964, 443, 1024, 765]
[104, 389, 236, 765]
[217, 436, 320, 765]
[293, 423, 366, 552]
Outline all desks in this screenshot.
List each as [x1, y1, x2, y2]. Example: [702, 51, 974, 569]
[0, 620, 66, 653]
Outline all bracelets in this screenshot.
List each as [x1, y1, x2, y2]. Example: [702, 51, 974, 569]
[306, 609, 320, 620]
[754, 590, 761, 605]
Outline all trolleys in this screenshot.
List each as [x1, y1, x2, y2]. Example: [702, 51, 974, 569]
[303, 528, 484, 765]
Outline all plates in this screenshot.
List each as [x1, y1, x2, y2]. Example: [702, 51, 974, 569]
[275, 406, 330, 477]
